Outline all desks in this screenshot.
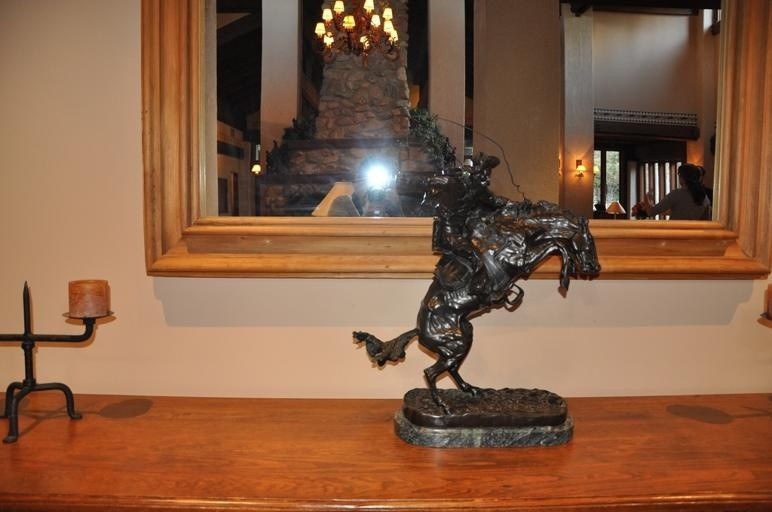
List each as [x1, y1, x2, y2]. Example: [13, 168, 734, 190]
[0, 392, 771, 512]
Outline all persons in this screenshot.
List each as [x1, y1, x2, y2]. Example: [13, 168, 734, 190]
[414, 154, 503, 294]
[328, 159, 404, 217]
[645, 163, 714, 220]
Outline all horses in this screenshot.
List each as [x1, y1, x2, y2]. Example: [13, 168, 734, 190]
[351, 199, 602, 416]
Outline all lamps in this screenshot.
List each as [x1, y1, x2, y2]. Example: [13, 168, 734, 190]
[608, 202, 626, 220]
[573, 158, 586, 176]
[251, 164, 262, 175]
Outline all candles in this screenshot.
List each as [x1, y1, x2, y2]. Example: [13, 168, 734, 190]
[67, 280, 113, 320]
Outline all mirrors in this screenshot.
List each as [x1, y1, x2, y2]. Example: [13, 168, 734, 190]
[140, 0, 772, 278]
